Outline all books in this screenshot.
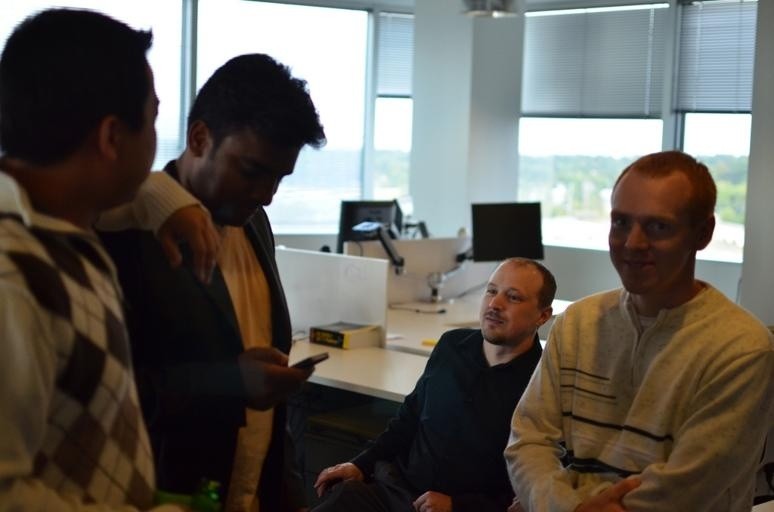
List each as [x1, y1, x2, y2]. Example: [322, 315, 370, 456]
[310, 321, 382, 350]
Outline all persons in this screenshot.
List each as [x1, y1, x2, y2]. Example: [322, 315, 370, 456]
[96, 54, 326, 512]
[311, 257, 557, 512]
[504, 151, 774, 512]
[0, 9, 220, 512]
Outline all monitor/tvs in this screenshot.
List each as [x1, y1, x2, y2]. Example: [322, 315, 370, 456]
[337, 201, 404, 267]
[472, 202, 543, 262]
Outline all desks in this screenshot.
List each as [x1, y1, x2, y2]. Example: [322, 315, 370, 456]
[343, 288, 573, 357]
[284, 336, 428, 405]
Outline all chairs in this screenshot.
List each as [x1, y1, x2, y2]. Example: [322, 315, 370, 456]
[325, 447, 508, 512]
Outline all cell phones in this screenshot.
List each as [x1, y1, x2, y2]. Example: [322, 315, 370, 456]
[290, 353, 328, 366]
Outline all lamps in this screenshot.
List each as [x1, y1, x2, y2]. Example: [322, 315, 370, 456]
[462, 0, 515, 20]
[523, 0, 670, 17]
